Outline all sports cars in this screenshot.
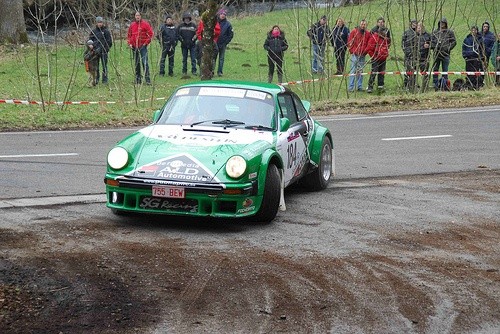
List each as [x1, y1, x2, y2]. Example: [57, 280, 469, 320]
[103, 78, 334, 223]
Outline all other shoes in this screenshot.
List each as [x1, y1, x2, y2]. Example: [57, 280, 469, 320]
[145, 77, 151, 85]
[160, 72, 174, 77]
[137, 77, 141, 84]
[366, 87, 372, 93]
[96, 81, 107, 84]
[86, 82, 97, 88]
[378, 88, 383, 93]
[182, 72, 224, 78]
[333, 71, 343, 75]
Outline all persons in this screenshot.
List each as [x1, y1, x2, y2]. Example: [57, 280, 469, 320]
[263, 25, 288, 84]
[461, 26, 484, 90]
[194, 7, 234, 76]
[127, 10, 153, 86]
[327, 18, 351, 75]
[177, 13, 198, 76]
[401, 18, 457, 93]
[306, 15, 335, 75]
[474, 21, 496, 82]
[156, 16, 179, 77]
[83, 40, 102, 87]
[348, 18, 371, 93]
[491, 31, 500, 88]
[90, 16, 112, 85]
[366, 17, 391, 94]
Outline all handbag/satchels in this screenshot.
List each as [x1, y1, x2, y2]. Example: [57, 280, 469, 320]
[437, 76, 451, 91]
[452, 79, 464, 91]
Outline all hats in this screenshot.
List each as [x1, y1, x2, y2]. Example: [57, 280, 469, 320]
[218, 8, 227, 15]
[86, 40, 94, 45]
[95, 16, 103, 21]
